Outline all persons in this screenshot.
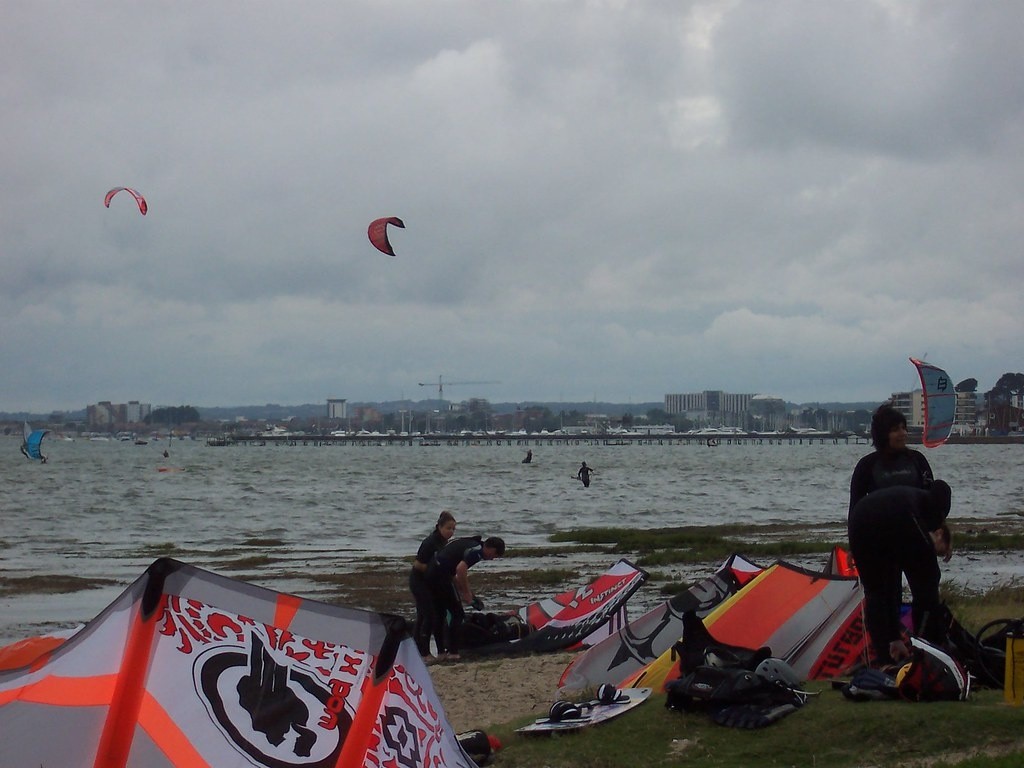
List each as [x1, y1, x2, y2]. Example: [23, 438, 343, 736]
[426, 535, 505, 661]
[528, 450, 533, 460]
[848, 406, 953, 663]
[578, 461, 593, 487]
[410, 512, 460, 665]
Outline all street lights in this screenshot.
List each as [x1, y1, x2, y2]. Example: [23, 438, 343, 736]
[398, 409, 408, 431]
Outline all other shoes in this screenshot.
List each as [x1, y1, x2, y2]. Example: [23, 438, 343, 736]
[423, 654, 437, 666]
[438, 650, 450, 662]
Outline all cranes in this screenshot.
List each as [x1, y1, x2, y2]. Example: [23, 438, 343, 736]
[417, 373, 505, 404]
